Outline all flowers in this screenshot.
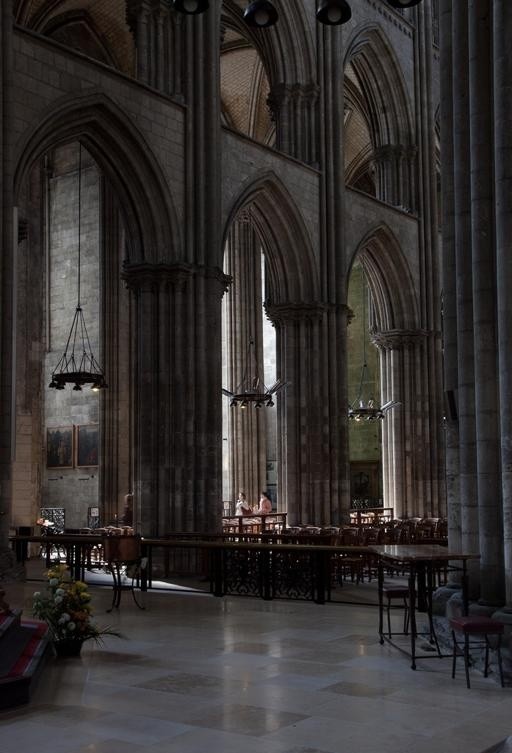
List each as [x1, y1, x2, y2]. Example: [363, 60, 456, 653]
[22, 558, 133, 650]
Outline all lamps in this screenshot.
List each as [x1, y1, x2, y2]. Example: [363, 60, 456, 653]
[47, 133, 109, 393]
[226, 220, 275, 410]
[349, 250, 385, 423]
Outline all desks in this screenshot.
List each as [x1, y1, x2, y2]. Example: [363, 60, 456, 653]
[364, 541, 482, 673]
[140, 539, 368, 604]
[8, 518, 140, 588]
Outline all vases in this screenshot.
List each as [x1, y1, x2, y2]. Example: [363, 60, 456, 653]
[54, 639, 84, 659]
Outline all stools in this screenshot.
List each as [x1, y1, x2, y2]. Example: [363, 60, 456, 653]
[449, 618, 506, 689]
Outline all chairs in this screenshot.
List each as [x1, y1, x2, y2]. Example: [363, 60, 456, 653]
[102, 536, 147, 612]
[203, 517, 449, 585]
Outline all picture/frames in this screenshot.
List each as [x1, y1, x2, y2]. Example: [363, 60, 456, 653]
[44, 424, 73, 470]
[75, 422, 98, 468]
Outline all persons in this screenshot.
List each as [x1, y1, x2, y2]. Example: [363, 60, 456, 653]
[260, 492, 273, 514]
[121, 493, 133, 528]
[39, 518, 49, 527]
[235, 493, 248, 516]
